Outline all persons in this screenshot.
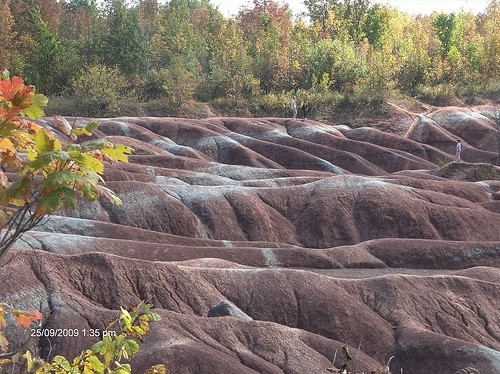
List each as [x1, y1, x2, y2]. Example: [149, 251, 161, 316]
[455, 139, 462, 163]
[301, 99, 308, 119]
[290, 95, 298, 120]
